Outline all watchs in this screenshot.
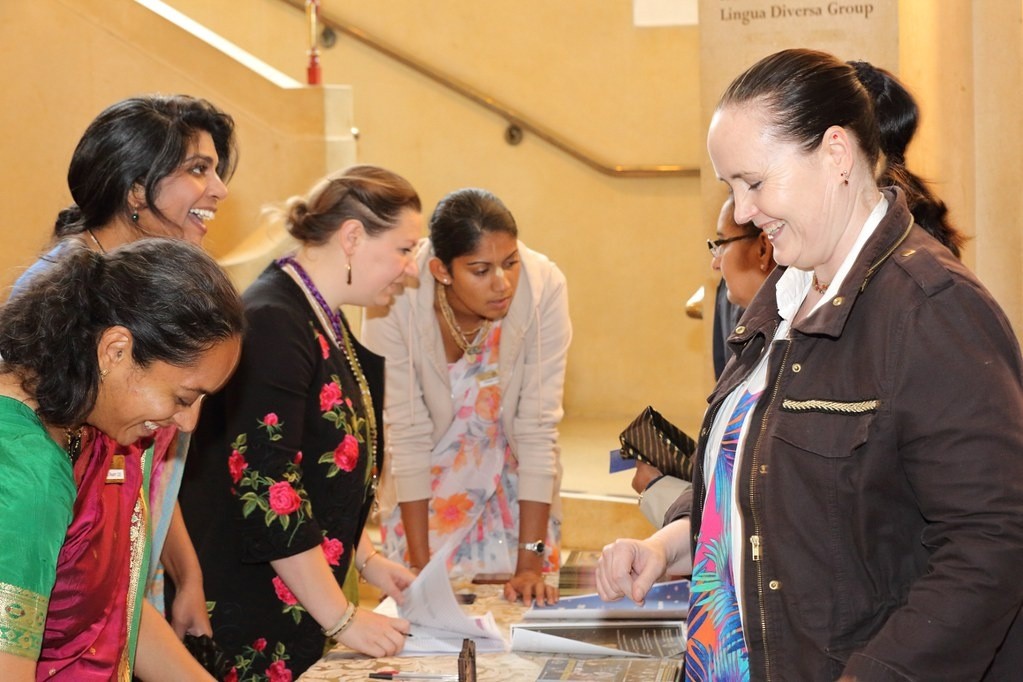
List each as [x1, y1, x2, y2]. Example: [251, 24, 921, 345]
[518, 540, 546, 555]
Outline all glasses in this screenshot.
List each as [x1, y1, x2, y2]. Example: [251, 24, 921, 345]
[707, 233, 758, 258]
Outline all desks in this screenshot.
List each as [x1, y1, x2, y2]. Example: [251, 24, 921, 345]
[296, 547, 688, 682]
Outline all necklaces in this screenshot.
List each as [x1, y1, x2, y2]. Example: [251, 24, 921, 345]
[88, 228, 110, 255]
[67, 425, 87, 461]
[330, 329, 383, 508]
[436, 283, 495, 363]
[812, 272, 829, 296]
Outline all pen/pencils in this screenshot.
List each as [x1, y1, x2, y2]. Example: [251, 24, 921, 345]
[368, 670, 452, 680]
[401, 633, 413, 637]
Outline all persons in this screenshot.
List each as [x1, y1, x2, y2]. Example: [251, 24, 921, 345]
[359, 186, 574, 606]
[162, 165, 421, 682]
[0, 244, 246, 682]
[9, 94, 238, 681]
[598, 47, 1023, 682]
